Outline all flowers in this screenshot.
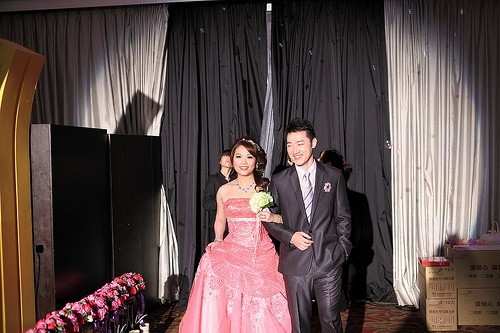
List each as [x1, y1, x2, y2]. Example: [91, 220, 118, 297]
[248, 192, 274, 214]
[323, 182, 331, 193]
[26, 272, 146, 333]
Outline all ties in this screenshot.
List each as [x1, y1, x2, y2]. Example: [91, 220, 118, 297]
[302, 172, 314, 224]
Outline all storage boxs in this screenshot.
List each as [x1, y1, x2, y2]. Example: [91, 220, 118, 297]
[417, 243, 500, 332]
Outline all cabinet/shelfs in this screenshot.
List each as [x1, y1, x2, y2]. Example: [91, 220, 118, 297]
[105, 133, 159, 322]
[30, 123, 113, 323]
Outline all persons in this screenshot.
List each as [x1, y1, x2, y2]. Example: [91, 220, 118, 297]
[179, 136, 293, 333]
[204, 149, 238, 244]
[262, 118, 351, 333]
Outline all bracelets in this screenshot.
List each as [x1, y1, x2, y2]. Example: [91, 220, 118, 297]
[278, 214, 282, 223]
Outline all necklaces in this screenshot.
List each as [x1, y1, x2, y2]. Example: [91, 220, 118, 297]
[236, 182, 255, 193]
